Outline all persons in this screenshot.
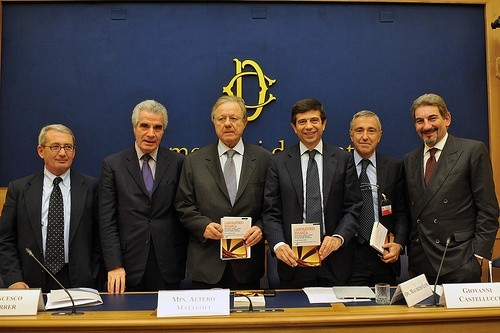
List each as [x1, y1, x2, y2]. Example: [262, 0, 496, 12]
[403, 94, 500, 285]
[0, 123, 105, 293]
[173, 96, 276, 290]
[261, 99, 365, 289]
[95, 100, 183, 295]
[348, 110, 412, 287]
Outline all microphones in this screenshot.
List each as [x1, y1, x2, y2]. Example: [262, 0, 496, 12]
[414, 238, 451, 308]
[25, 248, 84, 316]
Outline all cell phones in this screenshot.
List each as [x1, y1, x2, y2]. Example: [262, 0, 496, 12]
[263, 290, 276, 297]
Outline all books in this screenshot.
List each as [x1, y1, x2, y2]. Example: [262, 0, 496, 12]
[291, 223, 321, 267]
[220, 217, 252, 260]
[234, 290, 265, 307]
[369, 221, 394, 255]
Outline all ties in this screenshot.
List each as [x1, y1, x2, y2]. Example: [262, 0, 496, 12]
[45, 177, 65, 275]
[224, 150, 238, 208]
[424, 148, 438, 186]
[305, 150, 324, 244]
[142, 154, 154, 203]
[358, 160, 375, 243]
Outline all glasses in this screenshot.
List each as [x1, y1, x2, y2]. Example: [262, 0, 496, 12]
[42, 143, 76, 152]
